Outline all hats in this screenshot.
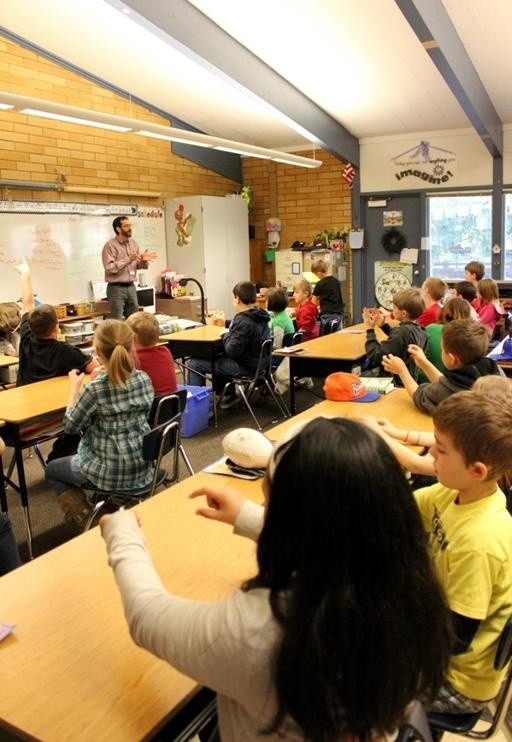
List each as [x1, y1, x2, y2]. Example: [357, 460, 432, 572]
[323, 371, 381, 403]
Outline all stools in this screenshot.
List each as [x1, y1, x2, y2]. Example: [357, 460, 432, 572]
[426, 614, 512, 739]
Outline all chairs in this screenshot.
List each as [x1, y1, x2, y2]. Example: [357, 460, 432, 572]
[83, 389, 194, 533]
[213, 318, 341, 431]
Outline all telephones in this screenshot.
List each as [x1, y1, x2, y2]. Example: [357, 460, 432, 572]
[291, 240, 305, 249]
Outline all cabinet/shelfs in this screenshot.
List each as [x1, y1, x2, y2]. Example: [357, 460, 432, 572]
[58, 311, 111, 346]
[156, 296, 208, 323]
[274, 248, 346, 313]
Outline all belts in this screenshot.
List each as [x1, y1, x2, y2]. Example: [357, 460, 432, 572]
[108, 281, 134, 287]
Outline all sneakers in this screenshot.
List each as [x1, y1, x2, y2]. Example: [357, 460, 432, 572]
[220, 393, 242, 409]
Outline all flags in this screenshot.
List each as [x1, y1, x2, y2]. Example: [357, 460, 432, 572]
[342, 163, 356, 184]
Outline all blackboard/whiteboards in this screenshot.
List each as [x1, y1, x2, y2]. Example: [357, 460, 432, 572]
[0, 209, 167, 313]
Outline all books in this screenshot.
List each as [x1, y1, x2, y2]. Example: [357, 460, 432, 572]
[19, 412, 66, 439]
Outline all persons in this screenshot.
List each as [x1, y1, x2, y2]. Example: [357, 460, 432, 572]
[100, 414, 456, 742]
[402, 391, 512, 741]
[44, 316, 155, 534]
[101, 217, 160, 319]
[0, 435, 21, 578]
[12, 258, 102, 387]
[92, 309, 180, 429]
[186, 257, 512, 512]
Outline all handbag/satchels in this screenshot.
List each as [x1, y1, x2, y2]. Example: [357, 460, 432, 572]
[1, 302, 23, 334]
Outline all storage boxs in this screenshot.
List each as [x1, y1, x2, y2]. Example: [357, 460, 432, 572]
[82, 331, 95, 342]
[70, 319, 95, 334]
[177, 384, 213, 437]
[64, 333, 83, 346]
[59, 323, 83, 334]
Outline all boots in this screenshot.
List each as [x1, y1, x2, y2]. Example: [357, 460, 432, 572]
[57, 488, 98, 532]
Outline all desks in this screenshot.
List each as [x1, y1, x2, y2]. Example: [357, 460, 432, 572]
[0, 354, 19, 390]
[256, 295, 313, 308]
[158, 323, 229, 428]
[0, 374, 91, 560]
[486, 334, 512, 369]
[0, 387, 434, 742]
[271, 314, 402, 416]
[95, 301, 110, 312]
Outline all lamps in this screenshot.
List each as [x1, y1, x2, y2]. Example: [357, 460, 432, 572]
[0, 91, 323, 169]
